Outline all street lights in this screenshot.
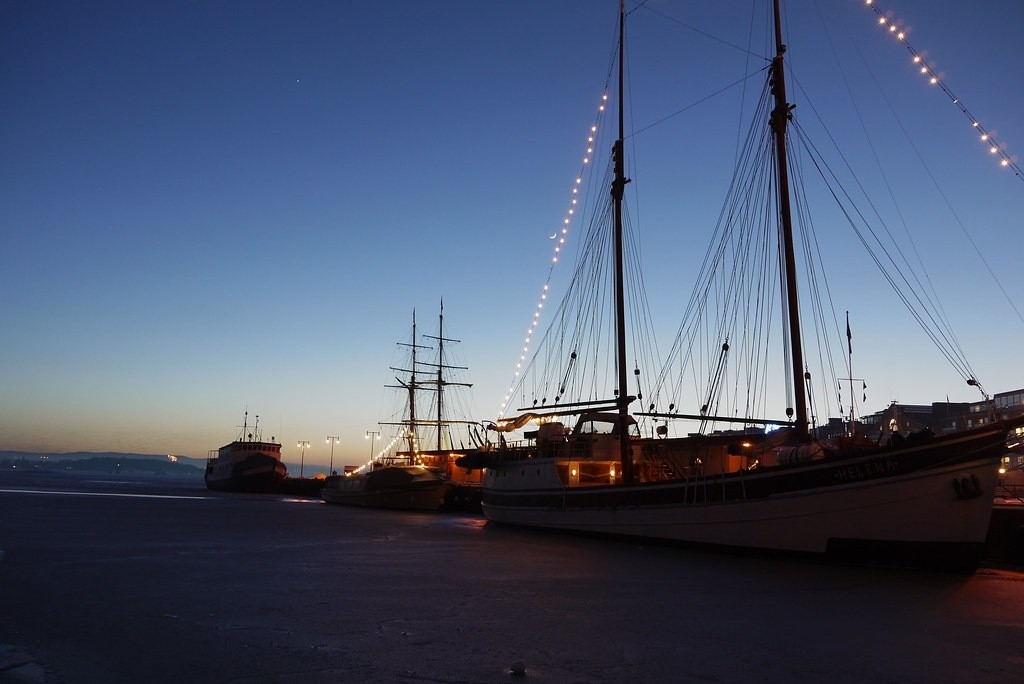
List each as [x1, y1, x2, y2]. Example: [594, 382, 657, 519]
[297, 440, 309, 477]
[326, 436, 340, 476]
[366, 431, 381, 472]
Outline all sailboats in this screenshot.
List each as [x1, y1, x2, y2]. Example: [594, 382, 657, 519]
[317, 1, 1024, 576]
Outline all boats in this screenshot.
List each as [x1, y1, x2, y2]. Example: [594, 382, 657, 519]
[205, 404, 289, 495]
